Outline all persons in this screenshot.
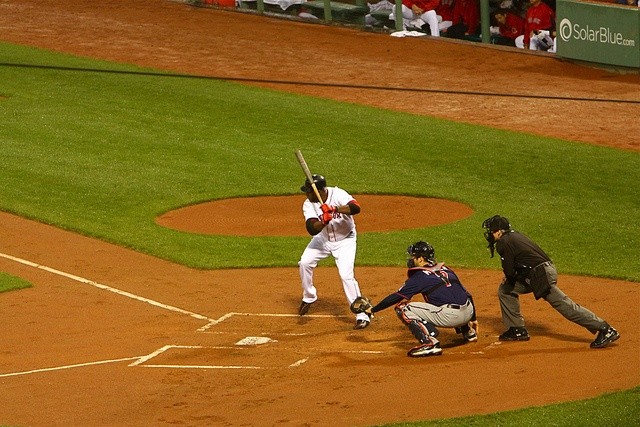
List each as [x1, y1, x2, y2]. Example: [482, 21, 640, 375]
[489, 10, 525, 48]
[447, 0, 480, 37]
[515, 1, 555, 50]
[393, 0, 440, 37]
[351, 240, 479, 356]
[297, 174, 371, 329]
[482, 215, 620, 349]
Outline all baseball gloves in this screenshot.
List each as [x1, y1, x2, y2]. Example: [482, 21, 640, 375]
[350, 296, 372, 313]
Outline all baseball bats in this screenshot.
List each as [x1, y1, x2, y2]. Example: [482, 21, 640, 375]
[294, 149, 324, 205]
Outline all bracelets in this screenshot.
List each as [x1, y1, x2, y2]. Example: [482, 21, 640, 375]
[334, 206, 339, 213]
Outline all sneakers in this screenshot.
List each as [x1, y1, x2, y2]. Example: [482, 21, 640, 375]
[590, 324, 620, 347]
[354, 320, 370, 329]
[408, 342, 441, 355]
[298, 296, 317, 315]
[463, 329, 477, 341]
[499, 327, 529, 341]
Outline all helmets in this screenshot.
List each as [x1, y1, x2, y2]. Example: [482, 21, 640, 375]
[482, 215, 509, 242]
[408, 241, 437, 265]
[301, 175, 326, 193]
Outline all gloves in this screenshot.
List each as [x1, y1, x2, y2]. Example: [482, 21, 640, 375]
[503, 279, 516, 292]
[321, 204, 336, 213]
[319, 213, 333, 223]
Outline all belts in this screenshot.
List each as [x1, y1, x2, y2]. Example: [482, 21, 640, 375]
[529, 260, 554, 271]
[450, 301, 471, 309]
